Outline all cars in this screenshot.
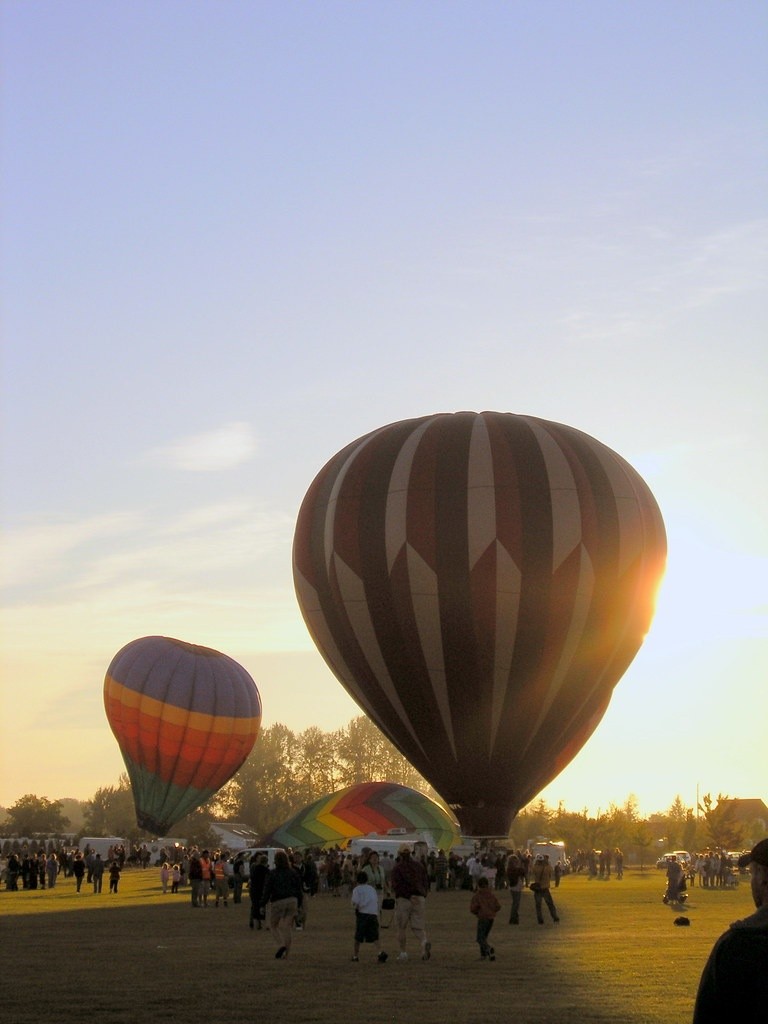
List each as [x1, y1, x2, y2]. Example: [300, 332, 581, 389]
[222, 847, 288, 888]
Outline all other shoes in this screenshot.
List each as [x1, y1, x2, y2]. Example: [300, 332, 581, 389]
[554, 917, 560, 924]
[274, 945, 285, 959]
[396, 951, 406, 960]
[216, 901, 219, 907]
[488, 947, 496, 961]
[377, 951, 388, 962]
[422, 942, 431, 961]
[223, 901, 227, 906]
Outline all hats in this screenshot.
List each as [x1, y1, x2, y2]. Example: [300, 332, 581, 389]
[737, 837, 768, 866]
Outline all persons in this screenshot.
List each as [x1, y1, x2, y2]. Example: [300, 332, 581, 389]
[4, 841, 153, 896]
[159, 842, 561, 930]
[390, 843, 432, 963]
[667, 856, 683, 905]
[693, 838, 768, 1023]
[261, 851, 305, 962]
[532, 854, 560, 925]
[690, 851, 737, 890]
[565, 848, 624, 878]
[350, 871, 389, 964]
[470, 877, 502, 962]
[507, 854, 528, 925]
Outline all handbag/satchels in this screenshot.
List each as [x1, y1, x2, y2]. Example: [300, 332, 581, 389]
[380, 897, 395, 909]
[529, 883, 539, 891]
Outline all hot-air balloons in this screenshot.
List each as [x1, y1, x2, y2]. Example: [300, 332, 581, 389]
[289, 411, 670, 865]
[248, 782, 461, 860]
[102, 636, 263, 867]
